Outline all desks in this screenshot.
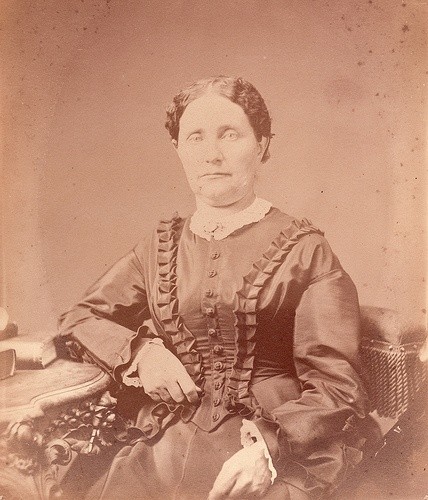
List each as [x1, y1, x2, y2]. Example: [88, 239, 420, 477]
[0, 358, 119, 500]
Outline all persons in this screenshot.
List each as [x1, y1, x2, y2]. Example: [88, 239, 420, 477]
[55, 73, 369, 500]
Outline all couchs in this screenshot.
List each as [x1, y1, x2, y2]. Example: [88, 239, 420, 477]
[352, 306, 427, 457]
